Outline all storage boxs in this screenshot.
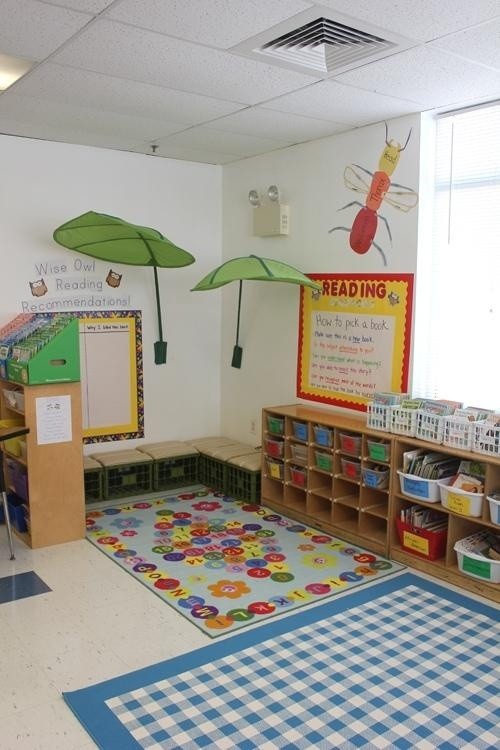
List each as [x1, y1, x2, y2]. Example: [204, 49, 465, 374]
[311, 423, 333, 448]
[337, 429, 362, 455]
[290, 442, 311, 466]
[486, 493, 498, 527]
[454, 537, 500, 584]
[396, 467, 454, 505]
[264, 414, 285, 436]
[262, 438, 285, 459]
[314, 450, 336, 474]
[290, 418, 308, 441]
[395, 513, 446, 564]
[339, 457, 361, 482]
[366, 437, 392, 464]
[288, 465, 309, 489]
[362, 464, 389, 490]
[263, 457, 285, 482]
[436, 476, 483, 520]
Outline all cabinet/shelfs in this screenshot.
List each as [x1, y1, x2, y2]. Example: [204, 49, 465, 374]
[0, 312, 87, 551]
[262, 402, 398, 561]
[388, 402, 500, 604]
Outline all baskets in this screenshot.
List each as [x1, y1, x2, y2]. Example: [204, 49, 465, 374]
[366, 401, 499, 459]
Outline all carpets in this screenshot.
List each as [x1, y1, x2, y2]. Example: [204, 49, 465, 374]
[61, 572, 500, 750]
[85, 486, 408, 639]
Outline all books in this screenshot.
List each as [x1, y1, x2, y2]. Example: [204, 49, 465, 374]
[475, 535, 500, 561]
[399, 503, 450, 534]
[0, 312, 76, 362]
[401, 448, 486, 495]
[368, 390, 500, 453]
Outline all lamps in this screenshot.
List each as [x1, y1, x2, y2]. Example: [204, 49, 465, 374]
[247, 184, 291, 238]
